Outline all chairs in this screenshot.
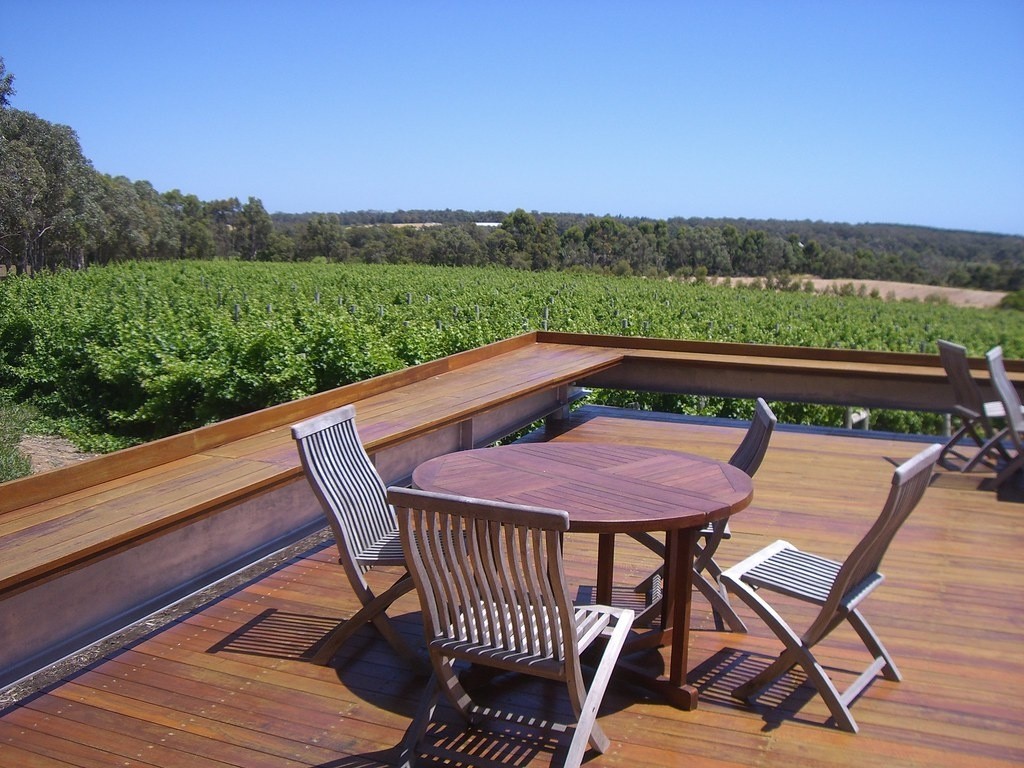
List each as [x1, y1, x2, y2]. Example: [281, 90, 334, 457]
[632, 398, 777, 632]
[720, 443, 943, 734]
[291, 404, 479, 674]
[936, 339, 1024, 474]
[985, 346, 1024, 491]
[386, 486, 636, 768]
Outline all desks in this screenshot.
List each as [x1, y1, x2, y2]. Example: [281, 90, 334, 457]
[414, 442, 754, 712]
